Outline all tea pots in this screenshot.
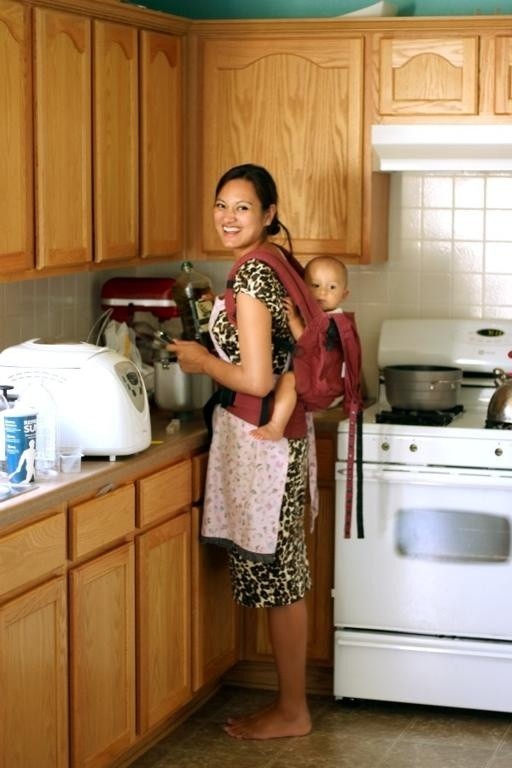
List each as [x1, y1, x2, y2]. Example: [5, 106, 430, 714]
[486, 379, 512, 424]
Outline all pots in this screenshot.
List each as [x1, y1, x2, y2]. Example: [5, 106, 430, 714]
[380, 364, 461, 411]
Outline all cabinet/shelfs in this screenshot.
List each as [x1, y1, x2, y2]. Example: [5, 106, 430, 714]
[1, 430, 242, 768]
[370, 15, 512, 124]
[229, 430, 336, 696]
[0, 0, 190, 283]
[192, 19, 388, 266]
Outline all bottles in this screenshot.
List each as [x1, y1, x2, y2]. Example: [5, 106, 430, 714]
[171, 261, 220, 353]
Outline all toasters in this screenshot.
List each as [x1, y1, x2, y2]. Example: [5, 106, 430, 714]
[0, 336, 154, 456]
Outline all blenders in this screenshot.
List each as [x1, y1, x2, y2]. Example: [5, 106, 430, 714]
[93, 277, 215, 413]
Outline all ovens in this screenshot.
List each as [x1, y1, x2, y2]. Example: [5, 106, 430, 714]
[327, 470, 512, 718]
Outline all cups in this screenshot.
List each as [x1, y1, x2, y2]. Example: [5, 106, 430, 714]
[3, 408, 35, 486]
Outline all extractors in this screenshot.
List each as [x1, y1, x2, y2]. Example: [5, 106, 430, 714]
[367, 122, 512, 174]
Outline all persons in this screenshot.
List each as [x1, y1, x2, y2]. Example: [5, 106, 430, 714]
[249, 251, 353, 441]
[165, 160, 322, 737]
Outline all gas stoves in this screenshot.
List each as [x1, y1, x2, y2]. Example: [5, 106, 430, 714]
[334, 401, 512, 470]
